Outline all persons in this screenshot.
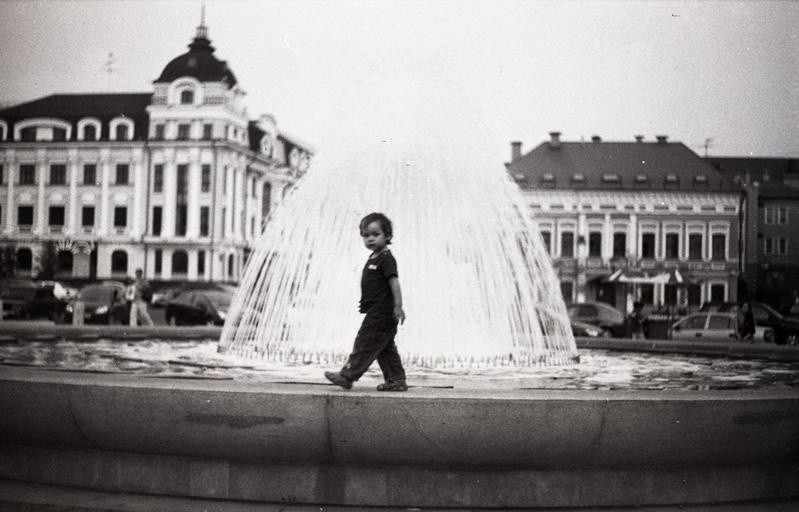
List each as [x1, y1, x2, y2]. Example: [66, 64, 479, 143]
[628, 301, 644, 340]
[128, 269, 154, 326]
[325, 213, 409, 392]
[734, 301, 756, 343]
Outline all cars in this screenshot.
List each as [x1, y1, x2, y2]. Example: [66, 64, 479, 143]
[566, 303, 628, 335]
[666, 310, 775, 346]
[64, 279, 131, 325]
[532, 302, 606, 337]
[165, 287, 261, 325]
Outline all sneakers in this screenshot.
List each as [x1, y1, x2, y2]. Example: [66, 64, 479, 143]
[377, 379, 408, 392]
[325, 371, 354, 389]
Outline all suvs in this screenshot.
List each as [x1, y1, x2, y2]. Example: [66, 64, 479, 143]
[700, 301, 798, 344]
